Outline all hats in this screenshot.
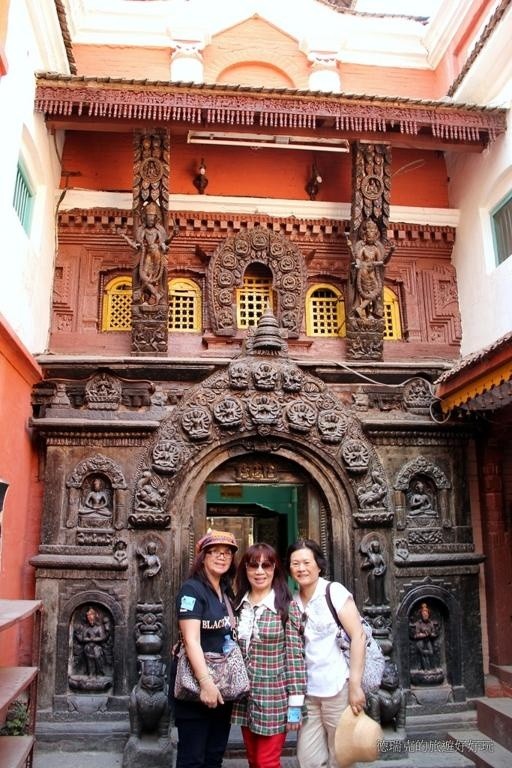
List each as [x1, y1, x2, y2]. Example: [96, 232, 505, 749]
[334, 704, 383, 768]
[195, 530, 239, 553]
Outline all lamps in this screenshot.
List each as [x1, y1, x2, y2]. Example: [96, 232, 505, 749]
[304, 158, 323, 200]
[193, 156, 209, 195]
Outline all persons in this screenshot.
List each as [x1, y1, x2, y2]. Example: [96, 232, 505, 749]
[345, 218, 397, 318]
[170, 529, 248, 768]
[408, 481, 437, 516]
[285, 539, 368, 768]
[409, 602, 441, 670]
[78, 478, 112, 517]
[120, 201, 181, 305]
[360, 540, 390, 605]
[74, 608, 107, 676]
[234, 542, 309, 768]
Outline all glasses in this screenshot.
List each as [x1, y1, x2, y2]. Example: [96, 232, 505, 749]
[205, 550, 233, 558]
[246, 561, 274, 572]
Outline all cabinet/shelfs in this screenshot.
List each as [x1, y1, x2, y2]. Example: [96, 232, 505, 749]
[1, 596, 46, 768]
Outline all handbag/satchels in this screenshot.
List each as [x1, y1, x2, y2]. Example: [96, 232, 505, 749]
[174, 639, 251, 701]
[325, 582, 385, 699]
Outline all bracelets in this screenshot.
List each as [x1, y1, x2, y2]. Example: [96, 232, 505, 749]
[287, 695, 306, 707]
[198, 675, 211, 684]
